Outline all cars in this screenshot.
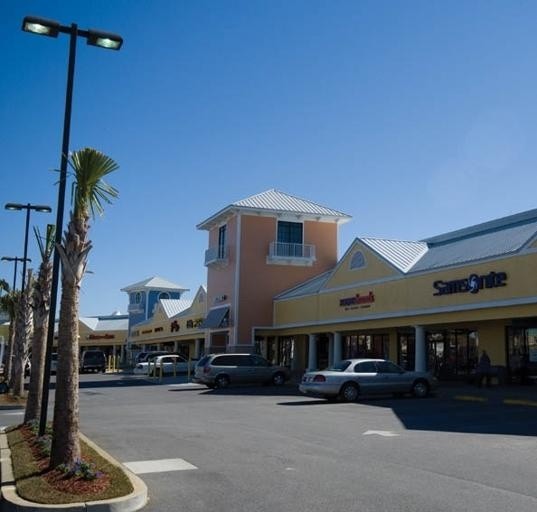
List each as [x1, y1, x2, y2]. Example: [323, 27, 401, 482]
[132, 351, 194, 376]
[299, 359, 436, 403]
[192, 353, 291, 389]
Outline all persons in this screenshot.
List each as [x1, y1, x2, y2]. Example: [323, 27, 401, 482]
[509, 349, 521, 385]
[350, 338, 383, 359]
[401, 339, 407, 354]
[427, 346, 476, 383]
[479, 350, 492, 388]
